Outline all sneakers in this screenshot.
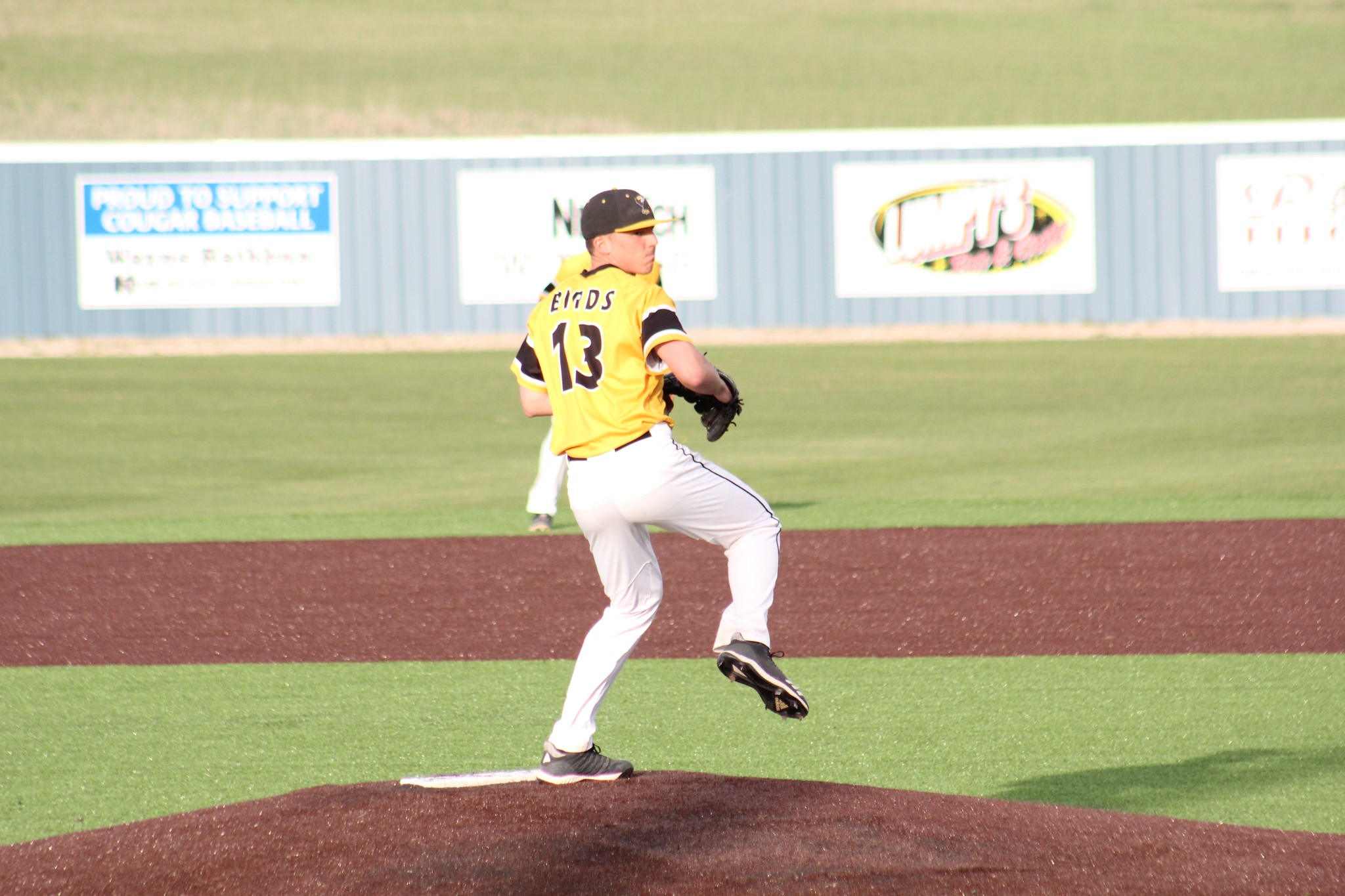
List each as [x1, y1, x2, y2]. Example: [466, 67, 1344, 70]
[537, 741, 634, 785]
[718, 639, 809, 720]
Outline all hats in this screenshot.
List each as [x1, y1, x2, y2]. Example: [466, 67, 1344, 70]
[581, 188, 679, 240]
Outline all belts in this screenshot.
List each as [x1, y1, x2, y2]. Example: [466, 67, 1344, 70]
[567, 430, 651, 461]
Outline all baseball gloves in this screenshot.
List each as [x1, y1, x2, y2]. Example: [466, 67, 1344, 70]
[663, 369, 745, 442]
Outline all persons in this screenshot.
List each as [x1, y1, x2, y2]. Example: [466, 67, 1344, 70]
[509, 188, 810, 785]
[525, 250, 666, 533]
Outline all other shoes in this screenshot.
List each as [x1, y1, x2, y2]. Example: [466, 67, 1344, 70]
[529, 514, 552, 531]
